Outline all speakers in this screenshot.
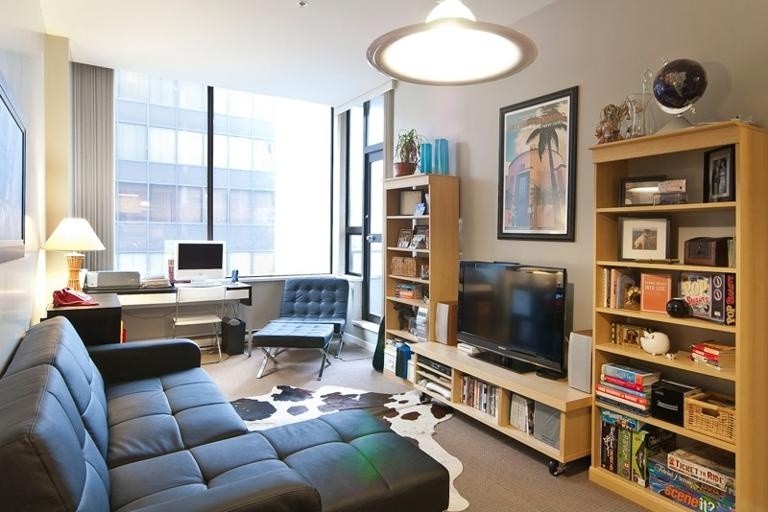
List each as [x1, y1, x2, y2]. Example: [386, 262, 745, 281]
[230, 270, 238, 283]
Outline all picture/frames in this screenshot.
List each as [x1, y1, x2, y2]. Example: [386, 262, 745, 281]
[703, 144, 736, 202]
[497, 85, 580, 243]
[0, 71, 26, 264]
[617, 216, 671, 262]
[618, 176, 668, 206]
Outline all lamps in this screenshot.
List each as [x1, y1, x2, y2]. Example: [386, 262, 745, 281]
[366, 0, 538, 87]
[41, 218, 106, 291]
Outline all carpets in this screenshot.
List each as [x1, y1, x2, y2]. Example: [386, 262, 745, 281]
[229, 385, 470, 512]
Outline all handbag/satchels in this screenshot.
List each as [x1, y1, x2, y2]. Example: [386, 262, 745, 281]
[392, 257, 423, 276]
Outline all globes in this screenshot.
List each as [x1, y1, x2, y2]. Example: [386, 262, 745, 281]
[649, 57, 709, 134]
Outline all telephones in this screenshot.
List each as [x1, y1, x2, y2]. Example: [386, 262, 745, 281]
[53, 287, 100, 308]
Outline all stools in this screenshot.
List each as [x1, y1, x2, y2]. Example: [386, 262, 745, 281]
[252, 324, 335, 381]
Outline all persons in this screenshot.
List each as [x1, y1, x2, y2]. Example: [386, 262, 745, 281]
[719, 160, 727, 193]
[712, 159, 721, 195]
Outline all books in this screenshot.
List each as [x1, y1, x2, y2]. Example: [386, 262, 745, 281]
[689, 341, 736, 373]
[610, 268, 631, 308]
[594, 362, 662, 417]
[460, 374, 498, 418]
[617, 273, 636, 308]
[603, 267, 611, 309]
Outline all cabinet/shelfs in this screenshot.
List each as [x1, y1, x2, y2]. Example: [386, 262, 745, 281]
[589, 120, 767, 512]
[409, 342, 593, 476]
[382, 174, 459, 349]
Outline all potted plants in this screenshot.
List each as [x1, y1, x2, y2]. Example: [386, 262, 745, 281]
[392, 129, 429, 177]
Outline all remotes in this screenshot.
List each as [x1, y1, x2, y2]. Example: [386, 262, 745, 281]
[493, 261, 519, 265]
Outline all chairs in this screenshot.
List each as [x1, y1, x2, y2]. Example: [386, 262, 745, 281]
[250, 277, 350, 359]
[172, 284, 226, 359]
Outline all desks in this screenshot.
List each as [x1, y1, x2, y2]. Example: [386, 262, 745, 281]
[46, 293, 122, 346]
[117, 276, 252, 349]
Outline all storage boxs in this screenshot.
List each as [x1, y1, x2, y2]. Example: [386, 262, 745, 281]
[647, 448, 736, 508]
[631, 428, 676, 488]
[651, 379, 697, 428]
[667, 441, 736, 496]
[617, 427, 631, 481]
[600, 420, 617, 474]
[649, 472, 736, 512]
[600, 407, 646, 434]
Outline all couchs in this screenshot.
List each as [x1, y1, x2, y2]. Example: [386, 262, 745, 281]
[0, 315, 450, 512]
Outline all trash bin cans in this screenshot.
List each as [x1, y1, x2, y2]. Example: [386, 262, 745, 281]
[220, 315, 246, 356]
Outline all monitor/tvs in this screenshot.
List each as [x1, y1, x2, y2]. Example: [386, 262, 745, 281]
[456, 261, 567, 374]
[174, 240, 225, 288]
